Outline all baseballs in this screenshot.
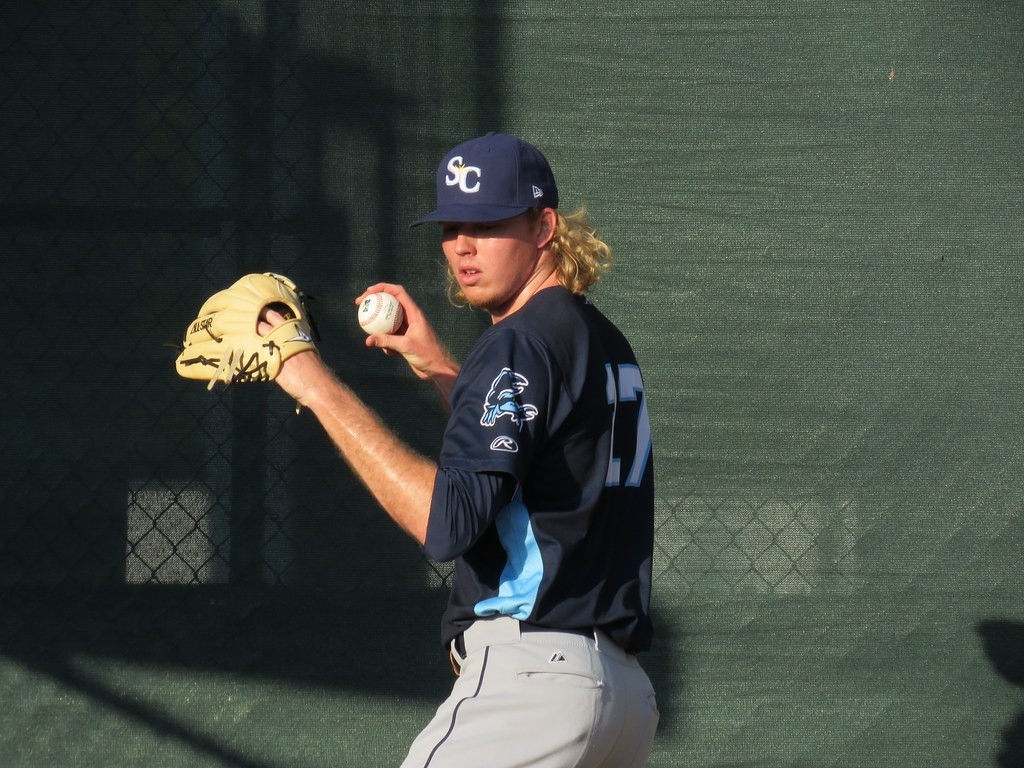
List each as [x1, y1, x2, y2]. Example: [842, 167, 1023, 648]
[357, 292, 403, 335]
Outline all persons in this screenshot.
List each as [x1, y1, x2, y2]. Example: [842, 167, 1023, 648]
[174, 130, 659, 768]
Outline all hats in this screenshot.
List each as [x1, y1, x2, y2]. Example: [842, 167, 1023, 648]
[409, 132, 559, 228]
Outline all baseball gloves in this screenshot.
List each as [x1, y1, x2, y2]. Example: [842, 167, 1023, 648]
[175, 271, 321, 391]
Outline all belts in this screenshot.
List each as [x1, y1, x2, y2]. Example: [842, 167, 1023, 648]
[450, 619, 636, 676]
